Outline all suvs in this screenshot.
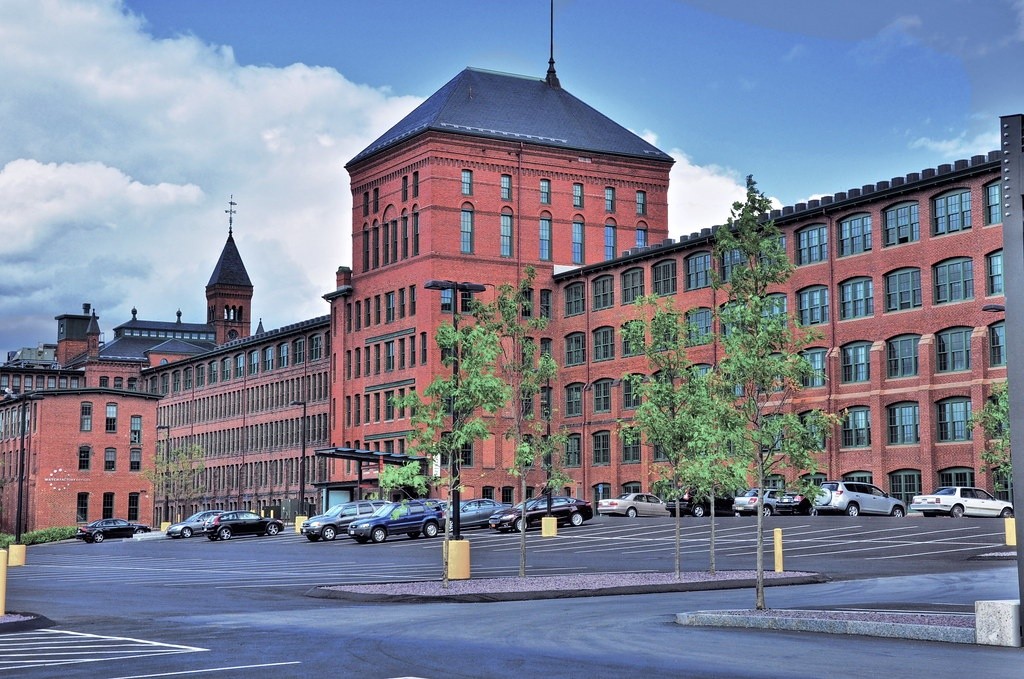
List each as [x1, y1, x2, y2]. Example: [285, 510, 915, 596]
[812, 480, 907, 517]
[300, 500, 393, 542]
[347, 498, 447, 543]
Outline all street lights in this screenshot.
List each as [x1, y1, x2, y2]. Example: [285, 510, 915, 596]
[531, 369, 557, 536]
[424, 279, 486, 579]
[290, 401, 308, 532]
[156, 425, 171, 531]
[4, 393, 45, 566]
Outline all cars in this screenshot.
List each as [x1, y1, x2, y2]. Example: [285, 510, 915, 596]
[597, 493, 671, 517]
[732, 488, 788, 516]
[166, 510, 225, 539]
[910, 486, 1014, 518]
[488, 496, 593, 532]
[438, 499, 514, 531]
[201, 510, 285, 540]
[775, 492, 814, 516]
[665, 485, 734, 517]
[76, 518, 152, 543]
